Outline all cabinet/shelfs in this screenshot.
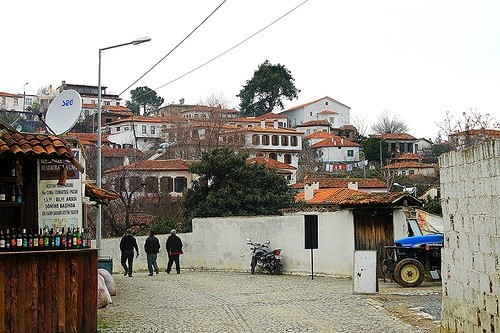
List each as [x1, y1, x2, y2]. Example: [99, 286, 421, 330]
[0, 176, 25, 207]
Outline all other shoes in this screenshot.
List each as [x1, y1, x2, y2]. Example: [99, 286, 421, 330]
[124, 271, 128, 276]
[129, 274, 132, 276]
[156, 268, 159, 274]
[165, 270, 170, 273]
[177, 271, 180, 274]
[148, 273, 153, 276]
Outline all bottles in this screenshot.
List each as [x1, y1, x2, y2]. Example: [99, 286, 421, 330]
[0, 225, 92, 251]
[17, 187, 22, 204]
[0, 183, 6, 201]
[11, 185, 16, 202]
[10, 160, 16, 177]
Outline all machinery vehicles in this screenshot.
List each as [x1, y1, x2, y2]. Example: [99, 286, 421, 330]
[383, 232, 444, 288]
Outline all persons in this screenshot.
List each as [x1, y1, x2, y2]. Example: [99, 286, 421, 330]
[120, 228, 139, 277]
[165, 229, 184, 275]
[144, 229, 161, 276]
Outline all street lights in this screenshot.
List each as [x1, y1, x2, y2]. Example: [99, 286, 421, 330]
[96, 35, 151, 259]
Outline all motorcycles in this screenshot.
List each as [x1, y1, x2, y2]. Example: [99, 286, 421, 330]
[246, 238, 283, 276]
